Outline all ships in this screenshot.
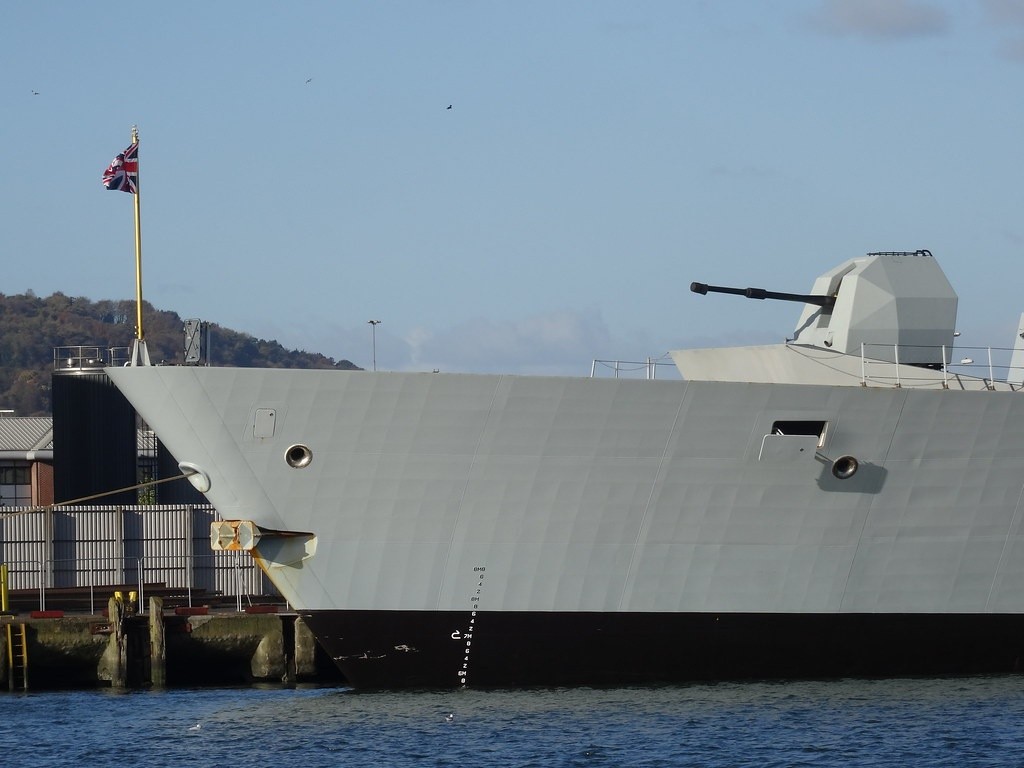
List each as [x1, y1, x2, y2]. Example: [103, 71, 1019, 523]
[99, 243, 1024, 694]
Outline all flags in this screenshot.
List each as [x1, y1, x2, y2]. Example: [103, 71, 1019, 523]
[102, 141, 139, 194]
